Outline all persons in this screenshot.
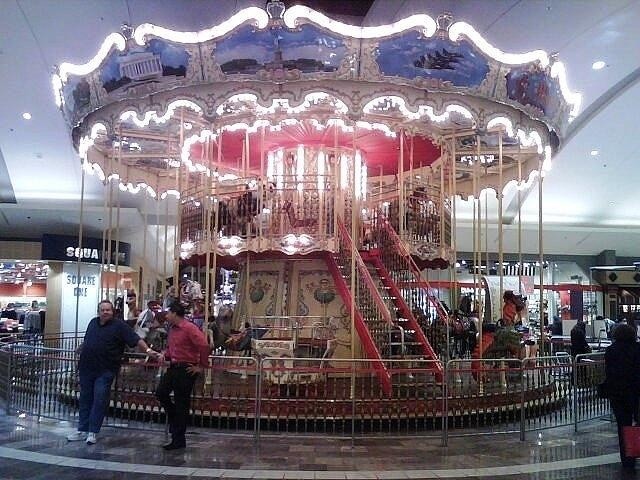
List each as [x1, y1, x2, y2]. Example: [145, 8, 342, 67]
[135, 301, 160, 352]
[66, 301, 159, 445]
[571, 320, 592, 386]
[610, 317, 628, 343]
[604, 324, 640, 470]
[552, 316, 562, 356]
[156, 303, 209, 450]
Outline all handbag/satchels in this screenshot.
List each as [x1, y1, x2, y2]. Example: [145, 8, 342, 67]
[622, 425, 640, 458]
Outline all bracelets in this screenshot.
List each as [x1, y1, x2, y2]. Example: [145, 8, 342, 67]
[146, 348, 151, 353]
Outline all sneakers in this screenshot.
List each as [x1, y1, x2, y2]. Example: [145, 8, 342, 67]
[86, 432, 97, 445]
[162, 438, 186, 451]
[67, 430, 87, 442]
